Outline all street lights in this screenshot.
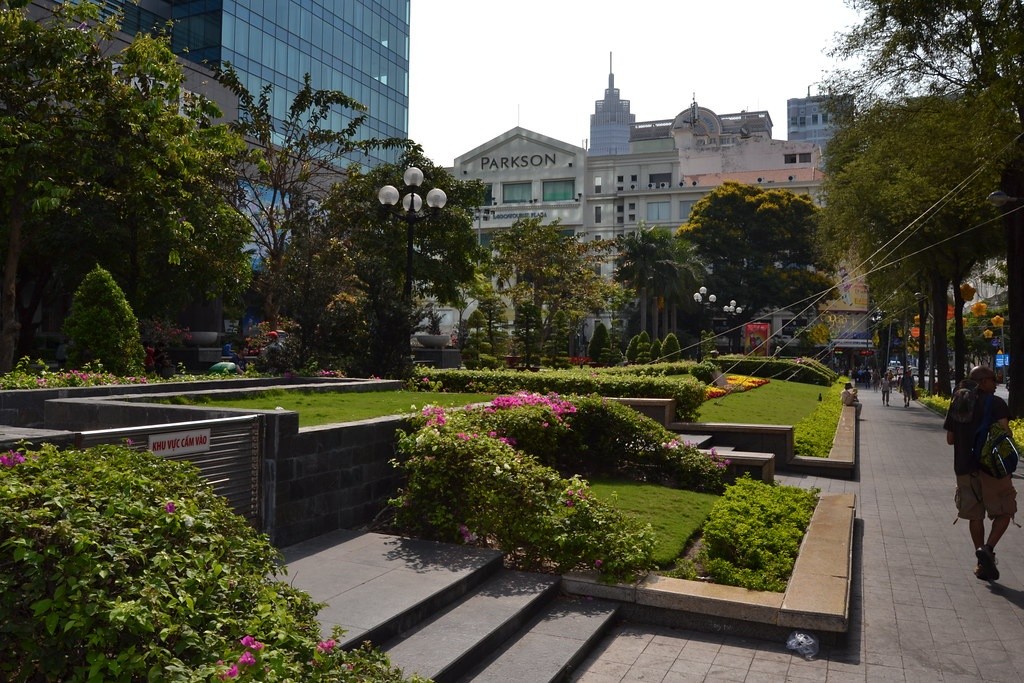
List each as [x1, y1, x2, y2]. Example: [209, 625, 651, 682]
[377, 166, 448, 367]
[723, 300, 742, 354]
[914, 292, 934, 399]
[693, 287, 717, 364]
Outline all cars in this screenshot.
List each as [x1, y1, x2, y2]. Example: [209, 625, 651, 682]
[887, 360, 974, 380]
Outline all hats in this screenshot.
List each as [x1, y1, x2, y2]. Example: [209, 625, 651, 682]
[844, 383, 853, 390]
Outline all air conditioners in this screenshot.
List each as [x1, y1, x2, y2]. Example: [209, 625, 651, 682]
[647, 183, 656, 188]
[756, 176, 768, 183]
[574, 193, 582, 202]
[691, 180, 700, 186]
[529, 198, 539, 204]
[630, 183, 639, 190]
[679, 182, 686, 187]
[788, 175, 797, 181]
[660, 182, 671, 188]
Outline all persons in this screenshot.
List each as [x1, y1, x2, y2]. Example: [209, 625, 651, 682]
[888, 369, 894, 393]
[222, 340, 234, 356]
[863, 367, 871, 389]
[872, 369, 880, 391]
[944, 366, 1014, 580]
[840, 382, 865, 421]
[231, 346, 251, 375]
[851, 367, 863, 384]
[879, 372, 893, 407]
[900, 369, 915, 408]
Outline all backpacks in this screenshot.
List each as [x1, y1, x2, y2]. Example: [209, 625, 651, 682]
[968, 394, 1020, 480]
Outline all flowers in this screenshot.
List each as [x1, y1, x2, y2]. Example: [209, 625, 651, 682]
[139, 318, 192, 364]
[243, 319, 287, 357]
[504, 335, 525, 355]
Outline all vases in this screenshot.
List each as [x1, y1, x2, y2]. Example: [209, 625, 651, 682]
[162, 365, 175, 378]
[244, 356, 257, 369]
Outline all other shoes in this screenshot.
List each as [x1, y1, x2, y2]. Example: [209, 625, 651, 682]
[974, 565, 989, 581]
[975, 545, 1000, 580]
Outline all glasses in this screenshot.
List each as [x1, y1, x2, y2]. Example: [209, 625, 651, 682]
[981, 376, 996, 382]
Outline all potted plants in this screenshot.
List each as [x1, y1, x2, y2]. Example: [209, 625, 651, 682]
[415, 312, 450, 345]
[504, 356, 523, 367]
